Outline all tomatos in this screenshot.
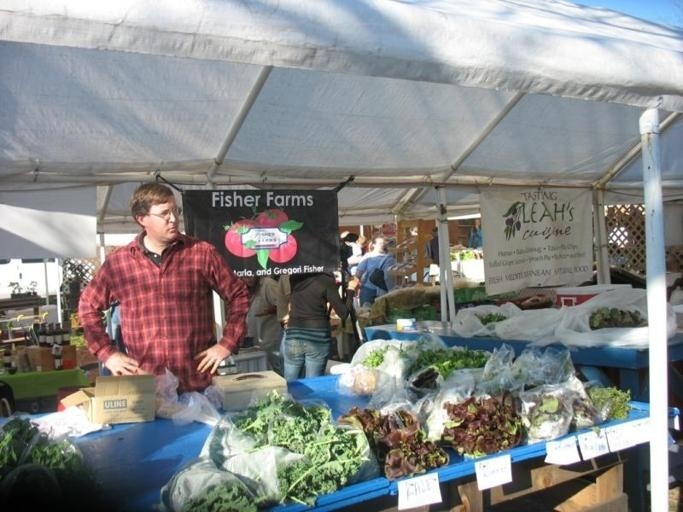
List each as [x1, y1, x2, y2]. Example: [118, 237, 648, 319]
[225, 208, 303, 269]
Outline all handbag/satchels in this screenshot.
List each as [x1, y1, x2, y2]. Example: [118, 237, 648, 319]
[369, 254, 396, 293]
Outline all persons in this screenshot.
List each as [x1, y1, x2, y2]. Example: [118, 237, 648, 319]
[467, 220, 482, 249]
[280, 228, 395, 380]
[242, 275, 287, 347]
[77, 182, 250, 395]
[423, 227, 439, 265]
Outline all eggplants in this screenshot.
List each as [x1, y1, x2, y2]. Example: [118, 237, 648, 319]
[409, 365, 440, 393]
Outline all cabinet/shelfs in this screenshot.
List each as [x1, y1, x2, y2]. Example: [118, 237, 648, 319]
[386, 216, 435, 285]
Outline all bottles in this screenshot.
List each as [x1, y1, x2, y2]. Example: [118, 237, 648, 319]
[50, 344, 63, 371]
[37, 323, 70, 346]
[0, 342, 16, 376]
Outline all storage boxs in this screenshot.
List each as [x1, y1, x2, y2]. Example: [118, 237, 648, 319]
[0, 342, 98, 373]
[61, 372, 159, 425]
[555, 283, 632, 308]
[212, 370, 292, 409]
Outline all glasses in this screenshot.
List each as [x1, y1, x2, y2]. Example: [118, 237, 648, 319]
[135, 205, 182, 218]
